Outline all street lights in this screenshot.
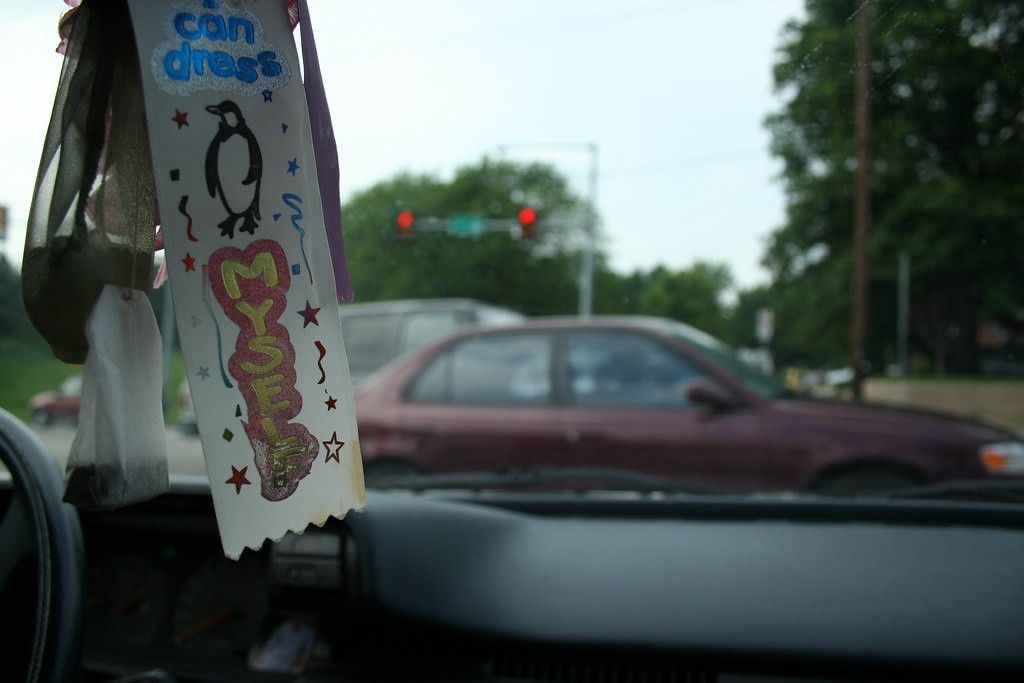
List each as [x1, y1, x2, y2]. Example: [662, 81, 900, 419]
[493, 140, 595, 321]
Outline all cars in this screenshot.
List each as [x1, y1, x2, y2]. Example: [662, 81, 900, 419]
[177, 294, 822, 437]
[28, 374, 90, 427]
[350, 322, 1024, 513]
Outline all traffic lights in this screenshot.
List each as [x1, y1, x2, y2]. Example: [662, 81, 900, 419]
[396, 206, 417, 241]
[517, 208, 539, 239]
